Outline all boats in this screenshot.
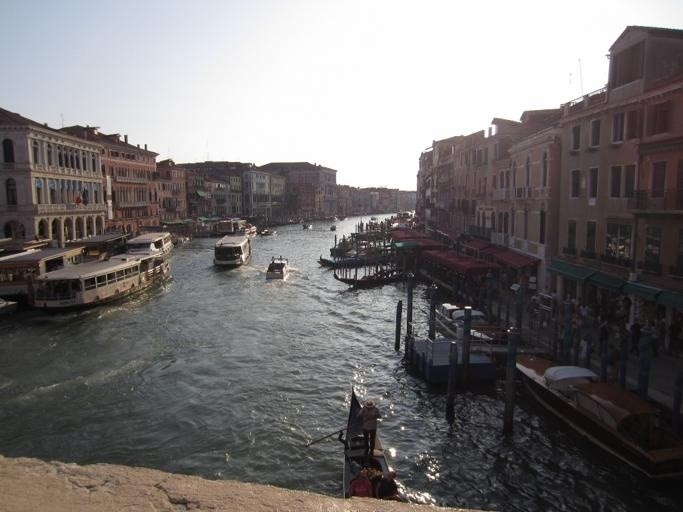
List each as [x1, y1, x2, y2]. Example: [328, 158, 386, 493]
[317, 212, 419, 290]
[266, 256, 288, 281]
[433, 302, 507, 345]
[262, 229, 279, 236]
[213, 235, 252, 269]
[303, 224, 312, 230]
[0, 231, 174, 319]
[343, 386, 404, 500]
[510, 350, 682, 480]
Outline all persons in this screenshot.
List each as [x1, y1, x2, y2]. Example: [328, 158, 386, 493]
[458, 252, 683, 370]
[374, 472, 396, 500]
[349, 469, 371, 497]
[354, 400, 380, 456]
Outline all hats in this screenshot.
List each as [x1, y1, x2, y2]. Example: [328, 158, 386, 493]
[363, 398, 376, 408]
